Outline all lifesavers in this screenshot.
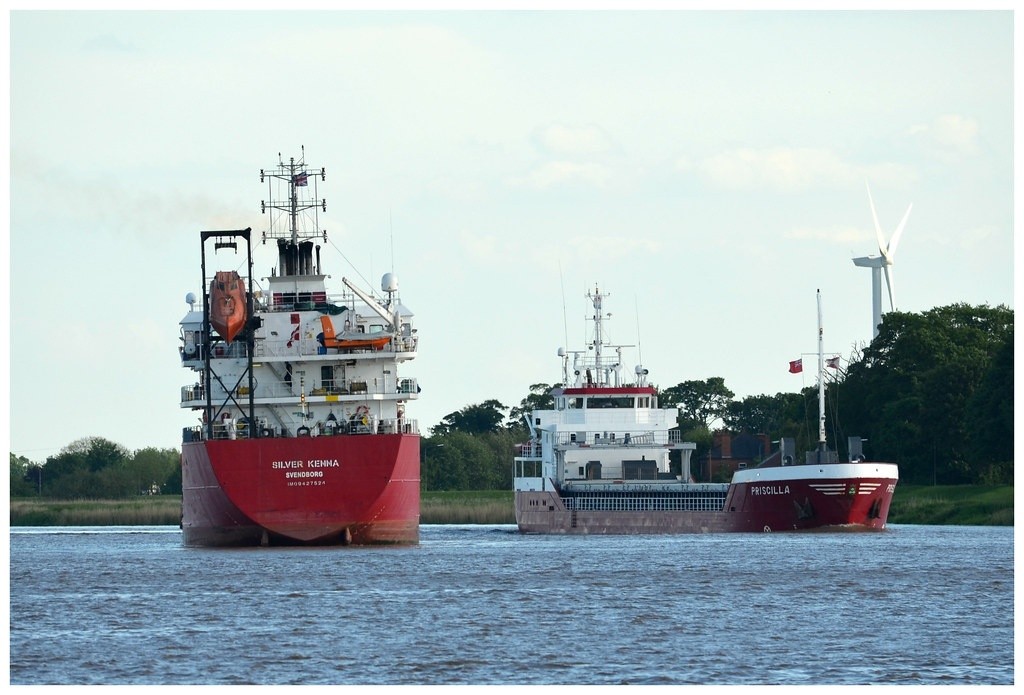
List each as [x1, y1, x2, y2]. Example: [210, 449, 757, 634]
[259, 428, 272, 438]
[297, 426, 310, 437]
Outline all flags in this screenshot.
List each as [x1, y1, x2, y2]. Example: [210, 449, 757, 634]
[788, 358, 802, 374]
[293, 170, 308, 186]
[291, 314, 300, 324]
[825, 357, 840, 369]
[287, 325, 299, 348]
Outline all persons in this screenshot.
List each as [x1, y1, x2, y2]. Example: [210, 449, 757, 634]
[193, 381, 201, 400]
[284, 362, 292, 388]
[397, 410, 404, 434]
[395, 378, 402, 394]
[527, 436, 538, 457]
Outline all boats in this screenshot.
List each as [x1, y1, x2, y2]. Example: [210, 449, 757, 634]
[176, 141, 421, 545]
[512, 279, 900, 534]
